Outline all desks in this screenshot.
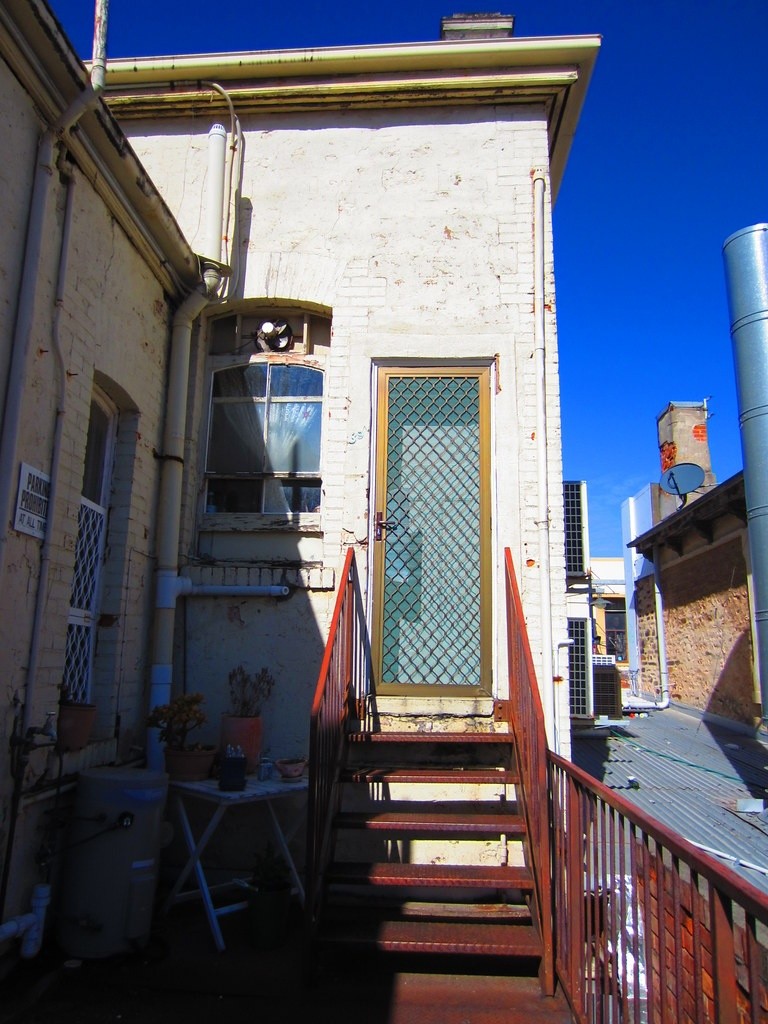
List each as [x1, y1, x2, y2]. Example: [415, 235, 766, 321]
[172, 765, 311, 951]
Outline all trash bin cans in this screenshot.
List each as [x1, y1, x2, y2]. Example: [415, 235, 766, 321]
[60, 765, 171, 959]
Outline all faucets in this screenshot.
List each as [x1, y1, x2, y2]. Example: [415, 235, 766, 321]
[26, 710, 59, 742]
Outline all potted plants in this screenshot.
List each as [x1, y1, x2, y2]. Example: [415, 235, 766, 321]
[220, 665, 278, 773]
[57, 682, 97, 750]
[246, 842, 296, 931]
[147, 692, 218, 775]
